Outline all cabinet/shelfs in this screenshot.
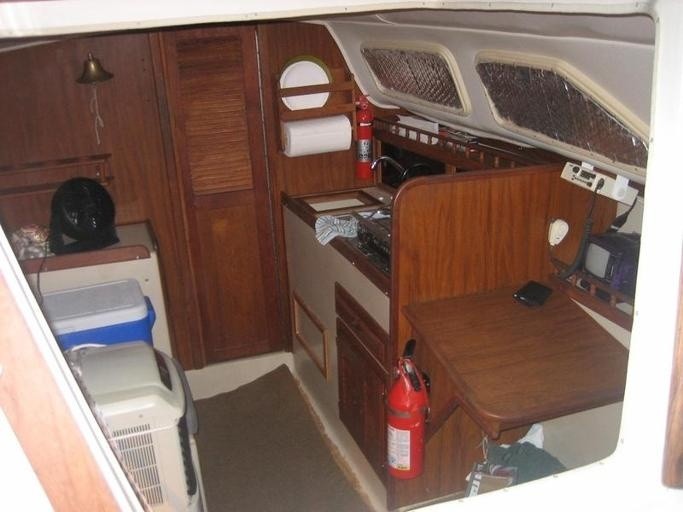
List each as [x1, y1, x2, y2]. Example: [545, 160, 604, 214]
[277, 206, 390, 511]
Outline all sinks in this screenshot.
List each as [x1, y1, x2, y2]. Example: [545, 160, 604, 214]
[300, 191, 386, 218]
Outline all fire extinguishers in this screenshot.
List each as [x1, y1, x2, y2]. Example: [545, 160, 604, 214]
[354, 94, 374, 179]
[386, 339, 432, 480]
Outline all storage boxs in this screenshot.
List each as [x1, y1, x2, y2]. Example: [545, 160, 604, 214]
[37, 280, 156, 356]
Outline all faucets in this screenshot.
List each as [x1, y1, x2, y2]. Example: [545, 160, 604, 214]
[371, 155, 408, 177]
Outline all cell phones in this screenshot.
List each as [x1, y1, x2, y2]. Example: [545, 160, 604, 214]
[513, 280, 553, 307]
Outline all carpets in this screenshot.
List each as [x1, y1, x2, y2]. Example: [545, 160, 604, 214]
[190, 360, 375, 512]
